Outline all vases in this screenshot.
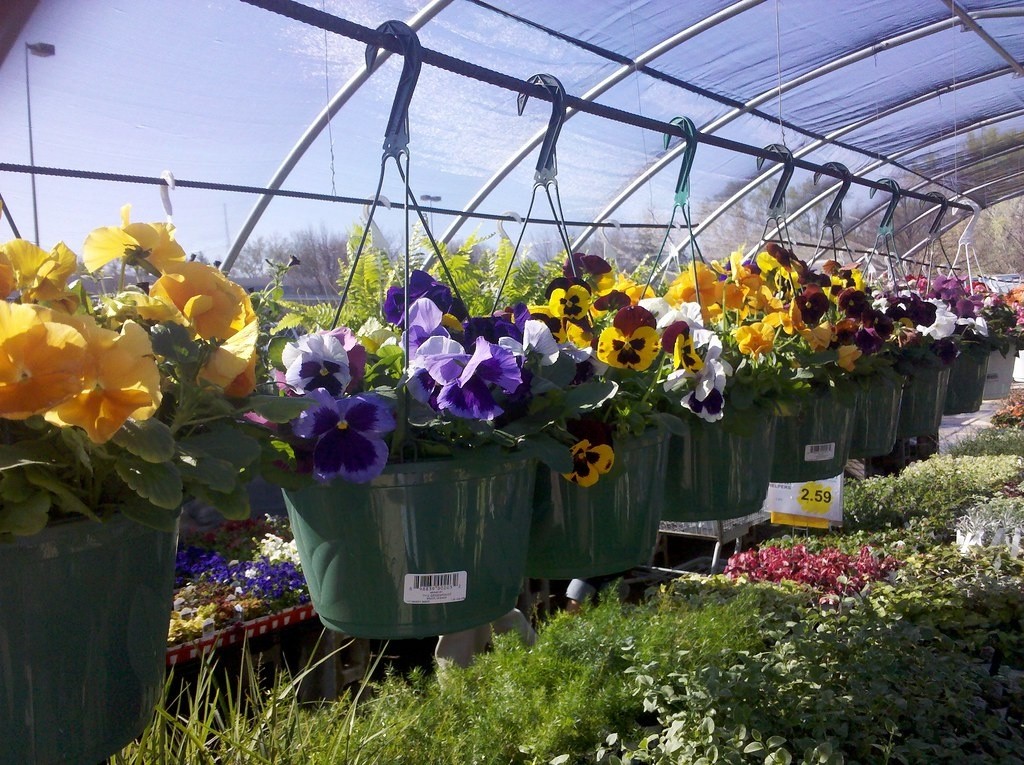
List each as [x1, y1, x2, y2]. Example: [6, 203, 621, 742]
[526, 434, 662, 580]
[901, 364, 950, 438]
[767, 393, 857, 484]
[281, 449, 536, 637]
[850, 380, 902, 461]
[0, 525, 179, 765]
[945, 348, 992, 419]
[664, 406, 769, 523]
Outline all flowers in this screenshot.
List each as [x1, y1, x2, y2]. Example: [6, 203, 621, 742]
[722, 545, 896, 590]
[2, 198, 1024, 496]
[167, 509, 309, 646]
